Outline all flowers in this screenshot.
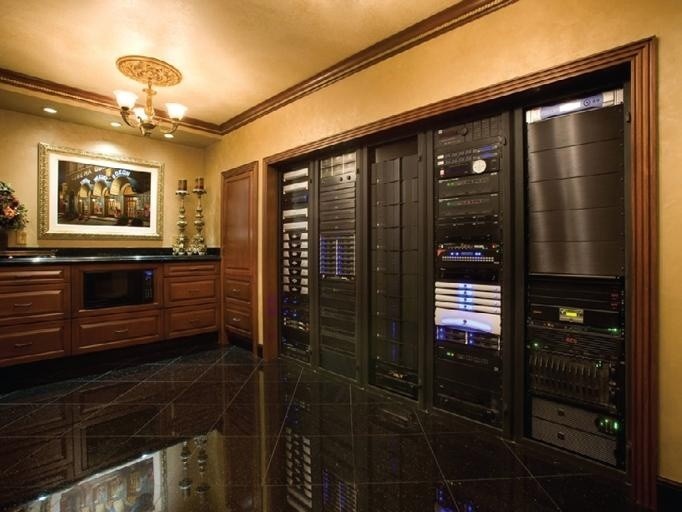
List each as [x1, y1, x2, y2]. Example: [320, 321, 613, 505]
[0, 181, 29, 232]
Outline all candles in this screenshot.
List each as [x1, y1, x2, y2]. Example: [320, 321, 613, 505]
[195, 178, 204, 189]
[181, 487, 191, 497]
[199, 494, 207, 502]
[177, 179, 187, 191]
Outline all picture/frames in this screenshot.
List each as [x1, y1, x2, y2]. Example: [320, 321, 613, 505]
[38, 142, 164, 241]
[39, 451, 168, 512]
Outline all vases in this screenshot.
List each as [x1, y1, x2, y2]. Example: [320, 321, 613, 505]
[0, 232, 8, 249]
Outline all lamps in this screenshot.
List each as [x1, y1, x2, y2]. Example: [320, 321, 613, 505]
[114, 56, 188, 137]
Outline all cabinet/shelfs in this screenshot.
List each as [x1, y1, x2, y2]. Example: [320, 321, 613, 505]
[223, 277, 257, 337]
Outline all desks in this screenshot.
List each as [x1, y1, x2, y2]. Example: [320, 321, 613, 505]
[0, 255, 222, 369]
